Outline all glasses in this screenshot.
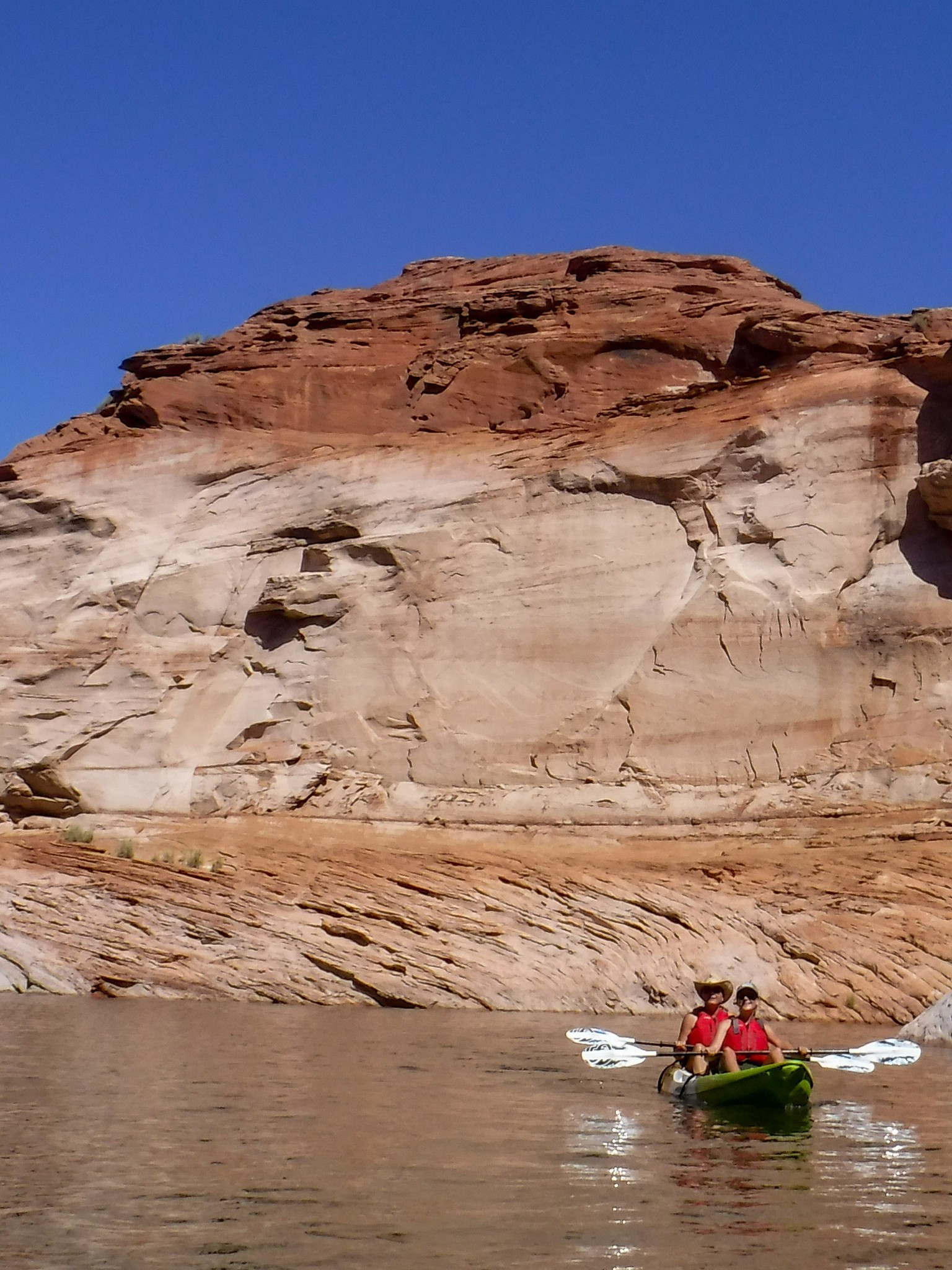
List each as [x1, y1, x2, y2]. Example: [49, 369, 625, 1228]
[702, 987, 722, 993]
[739, 989, 757, 1001]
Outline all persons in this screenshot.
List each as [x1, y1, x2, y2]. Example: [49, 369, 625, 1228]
[673, 972, 808, 1076]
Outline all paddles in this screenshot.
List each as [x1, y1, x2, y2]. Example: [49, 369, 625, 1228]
[581, 1038, 923, 1070]
[564, 1027, 874, 1074]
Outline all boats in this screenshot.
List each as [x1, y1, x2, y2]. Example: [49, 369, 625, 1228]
[671, 1059, 814, 1120]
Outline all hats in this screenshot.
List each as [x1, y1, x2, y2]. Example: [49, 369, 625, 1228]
[693, 978, 734, 1004]
[736, 984, 760, 998]
